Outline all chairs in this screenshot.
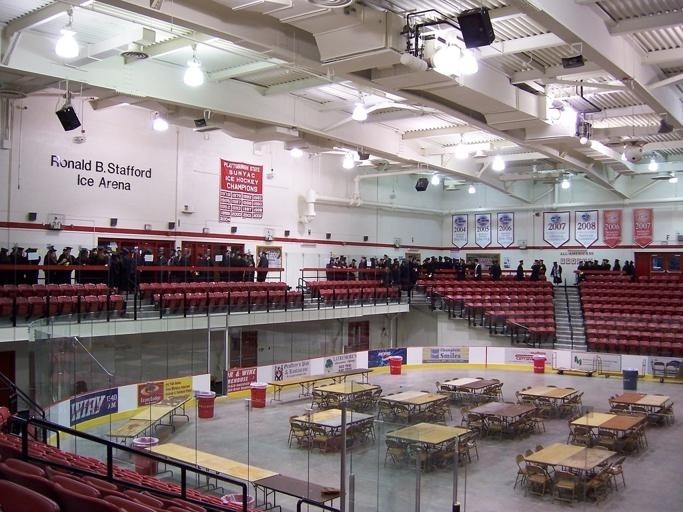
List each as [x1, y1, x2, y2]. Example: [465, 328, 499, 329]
[1, 407, 270, 512]
[0, 253, 404, 321]
[412, 267, 682, 354]
[286, 377, 674, 509]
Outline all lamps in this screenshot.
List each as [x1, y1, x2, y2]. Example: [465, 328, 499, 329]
[54, 3, 80, 62]
[149, 109, 168, 134]
[349, 88, 368, 122]
[54, 79, 82, 133]
[406, 6, 496, 61]
[180, 39, 206, 89]
[414, 177, 430, 192]
[577, 122, 593, 144]
[431, 44, 480, 84]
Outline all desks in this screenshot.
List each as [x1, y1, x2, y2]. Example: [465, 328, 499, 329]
[156, 395, 192, 432]
[305, 373, 346, 396]
[334, 367, 374, 383]
[269, 377, 317, 404]
[255, 474, 347, 512]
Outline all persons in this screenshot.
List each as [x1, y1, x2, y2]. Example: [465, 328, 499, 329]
[551, 262, 562, 286]
[516, 260, 524, 281]
[573, 259, 636, 285]
[0, 244, 268, 294]
[532, 260, 540, 281]
[539, 260, 546, 275]
[325, 255, 503, 291]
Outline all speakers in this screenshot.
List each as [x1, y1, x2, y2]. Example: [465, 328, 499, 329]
[55, 106, 81, 131]
[285, 231, 290, 236]
[458, 8, 496, 48]
[231, 227, 237, 233]
[326, 233, 331, 239]
[364, 236, 368, 241]
[28, 213, 36, 221]
[169, 222, 175, 229]
[415, 177, 429, 191]
[110, 218, 117, 226]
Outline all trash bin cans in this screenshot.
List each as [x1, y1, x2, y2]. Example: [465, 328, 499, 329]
[221, 494, 255, 511]
[197, 391, 216, 418]
[133, 437, 159, 475]
[250, 383, 268, 408]
[532, 357, 547, 373]
[623, 369, 638, 390]
[389, 356, 403, 375]
